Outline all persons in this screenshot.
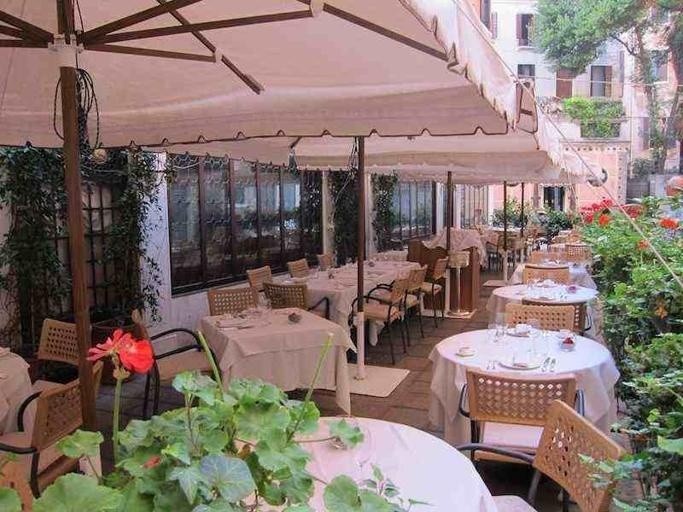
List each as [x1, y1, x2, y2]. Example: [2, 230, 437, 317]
[654, 175, 683, 239]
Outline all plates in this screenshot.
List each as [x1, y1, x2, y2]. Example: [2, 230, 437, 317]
[499, 352, 540, 369]
[456, 349, 474, 355]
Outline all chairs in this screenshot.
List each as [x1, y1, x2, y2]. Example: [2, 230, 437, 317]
[454, 400, 624, 512]
[317, 253, 333, 270]
[246, 265, 272, 292]
[486, 234, 516, 271]
[132, 310, 217, 418]
[0, 358, 103, 499]
[502, 304, 574, 332]
[351, 277, 409, 366]
[566, 243, 591, 265]
[285, 258, 309, 279]
[208, 288, 258, 315]
[456, 366, 583, 511]
[523, 299, 592, 335]
[379, 265, 424, 346]
[523, 264, 570, 285]
[261, 280, 329, 319]
[32, 318, 91, 367]
[416, 256, 450, 326]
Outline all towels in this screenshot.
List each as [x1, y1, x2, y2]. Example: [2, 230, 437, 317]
[513, 350, 527, 367]
[216, 318, 240, 327]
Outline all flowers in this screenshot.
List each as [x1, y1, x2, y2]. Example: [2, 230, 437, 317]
[0, 331, 429, 511]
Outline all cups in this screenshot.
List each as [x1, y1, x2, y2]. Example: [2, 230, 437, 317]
[525, 320, 539, 355]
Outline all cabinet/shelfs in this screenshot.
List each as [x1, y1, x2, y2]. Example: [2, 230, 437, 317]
[420, 227, 481, 312]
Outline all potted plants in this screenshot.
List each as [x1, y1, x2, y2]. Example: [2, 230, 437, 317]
[94, 154, 176, 383]
